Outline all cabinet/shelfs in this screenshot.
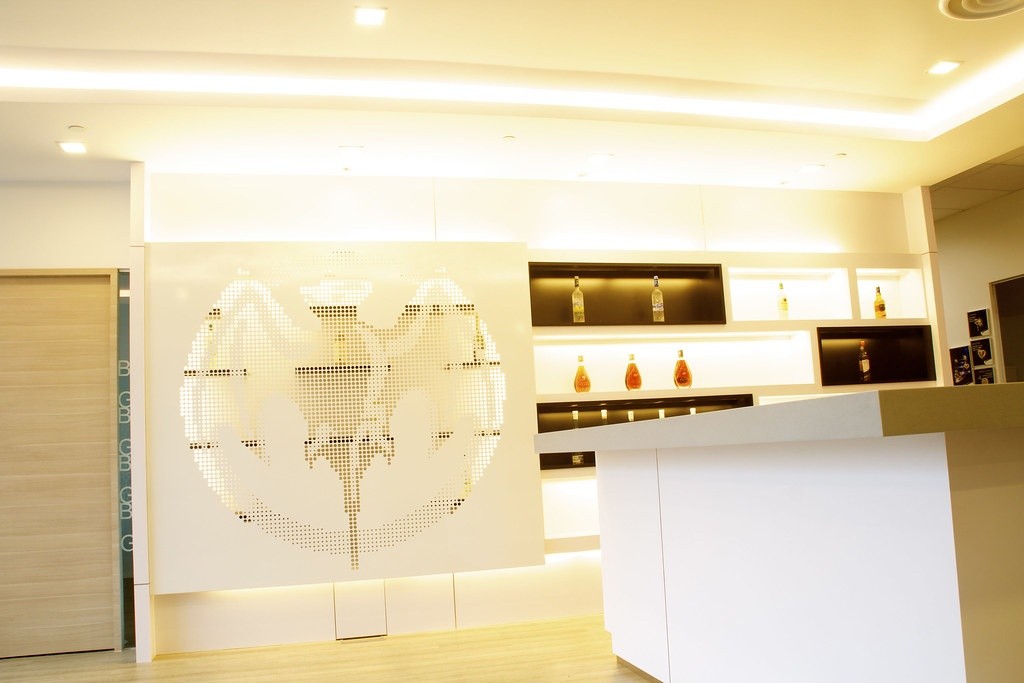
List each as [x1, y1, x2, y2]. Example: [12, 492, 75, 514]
[529, 251, 942, 555]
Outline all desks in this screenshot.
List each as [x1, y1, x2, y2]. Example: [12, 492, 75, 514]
[535, 382, 1024, 683]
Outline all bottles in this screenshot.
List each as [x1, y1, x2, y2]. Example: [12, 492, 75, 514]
[571, 411, 585, 466]
[858, 341, 870, 383]
[652, 276, 665, 323]
[574, 354, 591, 392]
[572, 276, 585, 323]
[599, 407, 696, 425]
[777, 282, 788, 320]
[673, 350, 692, 388]
[624, 353, 642, 390]
[873, 286, 887, 319]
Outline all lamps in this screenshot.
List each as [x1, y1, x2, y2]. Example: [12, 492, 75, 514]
[56, 126, 88, 154]
